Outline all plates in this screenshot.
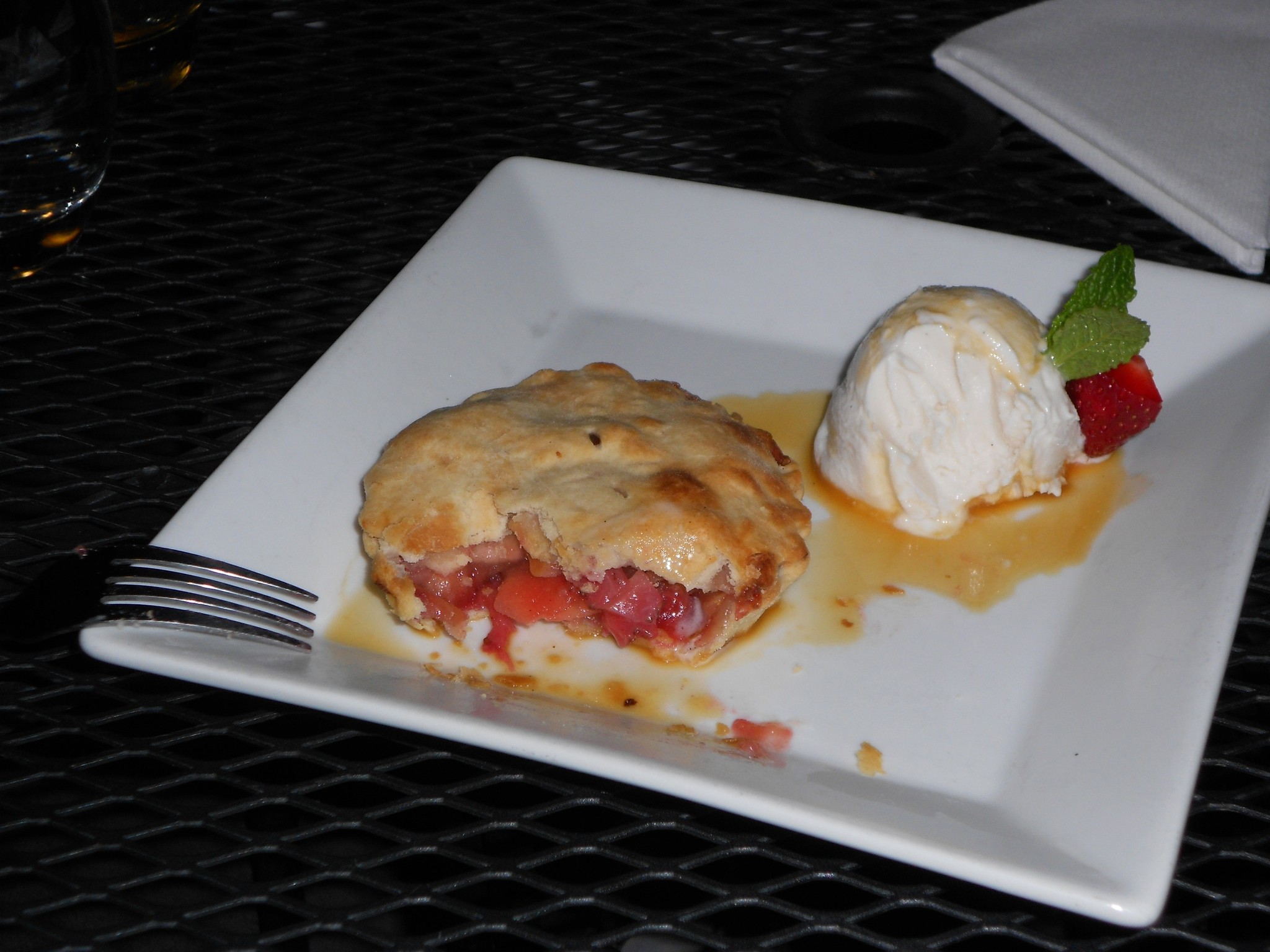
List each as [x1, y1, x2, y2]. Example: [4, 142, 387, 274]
[83, 156, 1269, 930]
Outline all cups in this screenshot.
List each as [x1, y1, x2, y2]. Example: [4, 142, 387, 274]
[0, 0, 201, 279]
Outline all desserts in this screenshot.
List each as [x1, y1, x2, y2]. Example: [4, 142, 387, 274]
[814, 242, 1162, 541]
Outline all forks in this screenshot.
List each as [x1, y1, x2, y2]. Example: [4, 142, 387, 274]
[0, 538, 318, 651]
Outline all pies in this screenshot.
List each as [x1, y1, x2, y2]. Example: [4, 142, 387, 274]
[359, 361, 810, 672]
